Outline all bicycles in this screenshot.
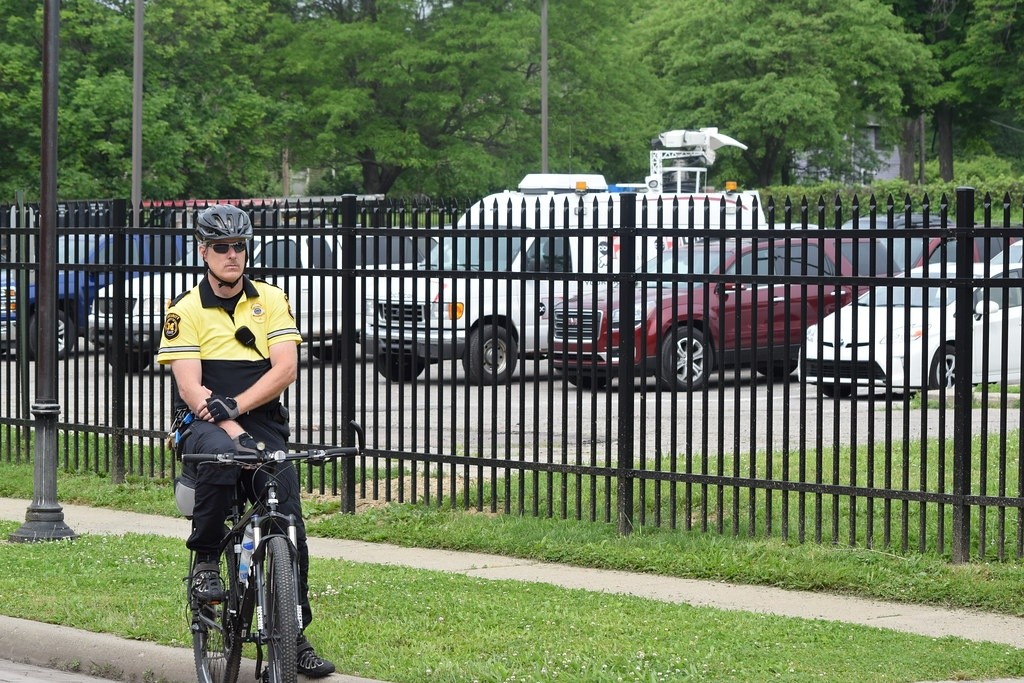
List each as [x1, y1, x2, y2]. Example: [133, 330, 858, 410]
[182, 420, 366, 683]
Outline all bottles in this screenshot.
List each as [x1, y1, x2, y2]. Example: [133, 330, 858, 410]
[238, 515, 261, 583]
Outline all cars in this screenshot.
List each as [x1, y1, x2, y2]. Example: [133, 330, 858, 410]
[0, 232, 187, 360]
[553, 213, 1023, 400]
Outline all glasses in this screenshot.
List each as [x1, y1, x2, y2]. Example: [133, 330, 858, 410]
[209, 241, 246, 253]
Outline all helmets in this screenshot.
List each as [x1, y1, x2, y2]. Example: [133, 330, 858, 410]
[194, 203, 253, 246]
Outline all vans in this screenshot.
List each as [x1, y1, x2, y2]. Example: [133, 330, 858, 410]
[365, 174, 766, 381]
[89, 234, 442, 373]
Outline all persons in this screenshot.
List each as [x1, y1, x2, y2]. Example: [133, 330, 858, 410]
[156, 204, 335, 678]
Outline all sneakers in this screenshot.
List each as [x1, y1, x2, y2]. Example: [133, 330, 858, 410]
[192, 550, 225, 606]
[296, 634, 335, 678]
